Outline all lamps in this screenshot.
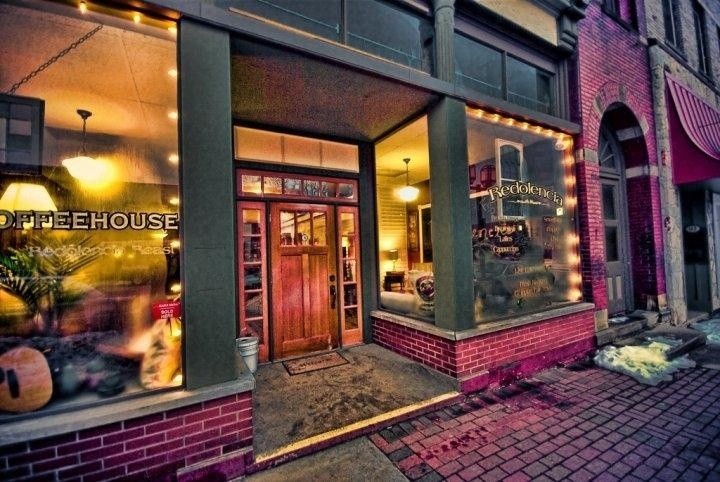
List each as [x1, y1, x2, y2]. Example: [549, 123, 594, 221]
[63, 109, 111, 182]
[398, 158, 419, 201]
[0, 183, 58, 252]
[388, 249, 398, 269]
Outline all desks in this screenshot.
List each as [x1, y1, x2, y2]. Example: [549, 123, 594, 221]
[385, 274, 406, 291]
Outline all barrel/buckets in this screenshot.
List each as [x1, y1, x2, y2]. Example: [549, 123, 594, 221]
[236, 337, 260, 374]
